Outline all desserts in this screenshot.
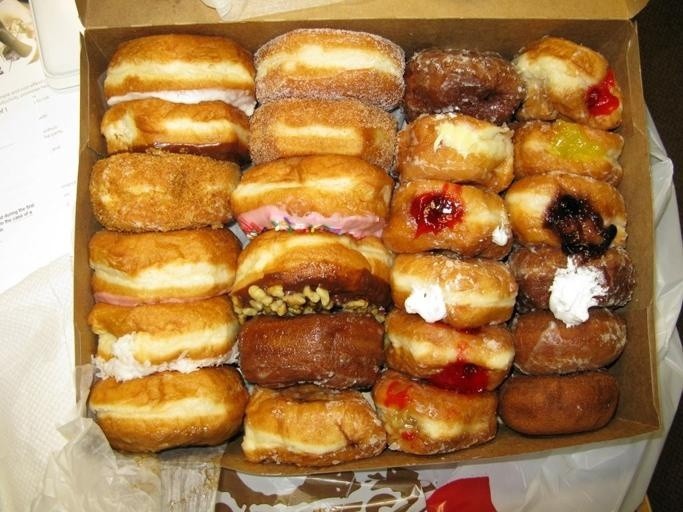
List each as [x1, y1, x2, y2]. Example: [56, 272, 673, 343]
[88, 28, 633, 467]
